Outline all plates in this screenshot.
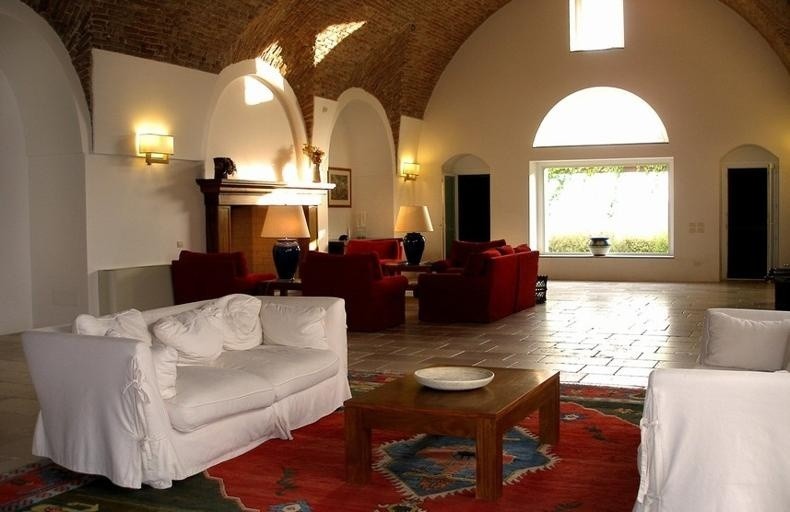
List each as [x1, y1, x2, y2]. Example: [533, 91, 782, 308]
[411, 365, 495, 392]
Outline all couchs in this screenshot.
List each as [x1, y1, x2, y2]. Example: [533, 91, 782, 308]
[18, 298, 352, 490]
[640, 305, 790, 512]
[418, 243, 540, 324]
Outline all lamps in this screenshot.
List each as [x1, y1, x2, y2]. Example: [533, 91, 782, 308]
[401, 164, 422, 180]
[395, 203, 435, 268]
[260, 204, 310, 281]
[138, 132, 175, 166]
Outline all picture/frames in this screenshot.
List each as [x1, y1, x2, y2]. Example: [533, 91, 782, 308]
[327, 166, 352, 209]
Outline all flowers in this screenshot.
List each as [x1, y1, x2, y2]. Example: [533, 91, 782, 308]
[302, 145, 325, 164]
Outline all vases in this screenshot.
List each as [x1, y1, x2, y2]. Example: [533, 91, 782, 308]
[314, 162, 321, 183]
[589, 237, 611, 257]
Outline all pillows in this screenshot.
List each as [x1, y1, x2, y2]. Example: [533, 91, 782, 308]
[70, 308, 153, 344]
[104, 328, 178, 397]
[153, 311, 224, 366]
[201, 290, 264, 351]
[697, 311, 788, 372]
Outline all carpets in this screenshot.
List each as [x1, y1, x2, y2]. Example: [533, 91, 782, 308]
[1, 370, 647, 512]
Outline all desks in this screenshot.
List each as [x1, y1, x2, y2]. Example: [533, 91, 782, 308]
[327, 237, 404, 254]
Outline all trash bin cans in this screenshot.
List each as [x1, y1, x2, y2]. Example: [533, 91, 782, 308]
[764, 267, 790, 311]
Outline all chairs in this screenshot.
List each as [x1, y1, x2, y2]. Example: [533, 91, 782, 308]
[433, 237, 505, 271]
[344, 237, 402, 263]
[169, 248, 277, 305]
[299, 251, 408, 331]
